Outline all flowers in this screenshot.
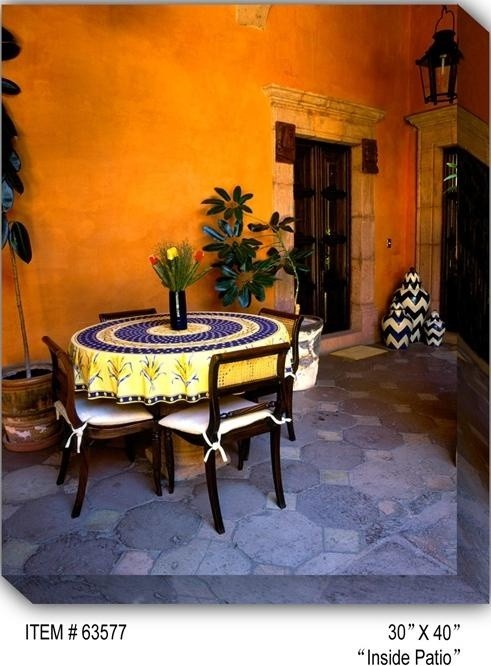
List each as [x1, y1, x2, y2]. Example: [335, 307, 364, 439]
[149, 237, 214, 296]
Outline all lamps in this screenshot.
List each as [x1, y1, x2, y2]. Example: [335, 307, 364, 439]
[414, 5, 465, 107]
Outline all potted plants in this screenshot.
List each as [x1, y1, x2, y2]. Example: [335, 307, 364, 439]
[198, 183, 325, 394]
[1, 24, 64, 453]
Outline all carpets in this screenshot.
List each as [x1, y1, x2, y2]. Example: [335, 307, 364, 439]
[330, 345, 390, 362]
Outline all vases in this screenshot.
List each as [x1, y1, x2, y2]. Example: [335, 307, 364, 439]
[168, 289, 188, 330]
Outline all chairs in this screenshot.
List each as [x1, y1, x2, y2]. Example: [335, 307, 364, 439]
[42, 305, 305, 537]
